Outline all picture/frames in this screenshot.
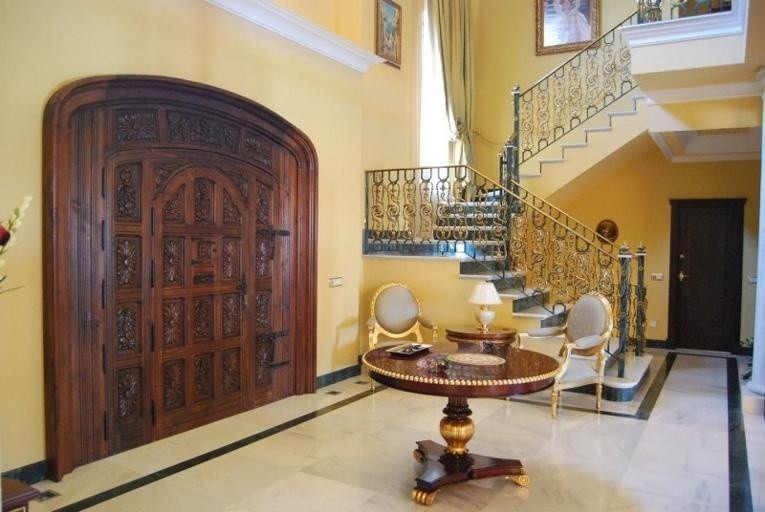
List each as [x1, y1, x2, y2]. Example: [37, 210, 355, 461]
[536, 0, 602, 54]
[377, 0, 402, 70]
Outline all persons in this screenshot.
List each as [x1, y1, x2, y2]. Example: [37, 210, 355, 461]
[551, 2, 592, 46]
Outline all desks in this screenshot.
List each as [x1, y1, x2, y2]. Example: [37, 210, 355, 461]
[360, 323, 561, 506]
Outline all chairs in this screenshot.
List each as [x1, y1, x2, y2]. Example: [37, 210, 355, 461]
[366, 281, 614, 419]
